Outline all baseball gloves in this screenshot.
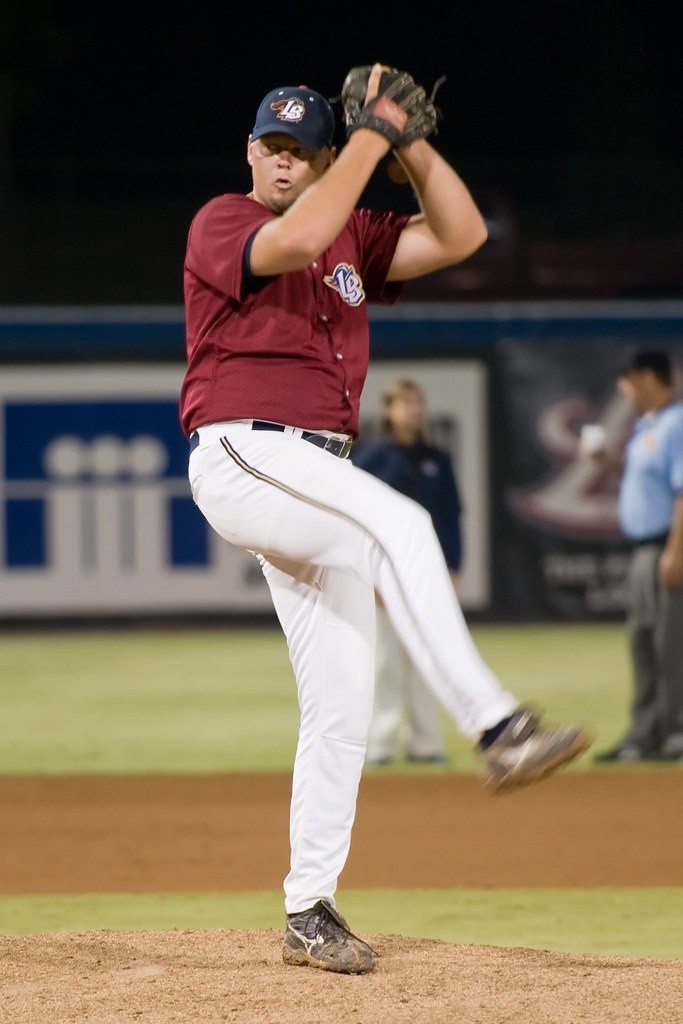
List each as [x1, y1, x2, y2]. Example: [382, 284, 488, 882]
[342, 63, 438, 150]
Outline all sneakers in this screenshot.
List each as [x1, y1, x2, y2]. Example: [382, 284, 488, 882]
[479, 708, 595, 796]
[282, 901, 380, 974]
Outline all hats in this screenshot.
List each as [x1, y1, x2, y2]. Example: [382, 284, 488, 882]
[251, 85, 337, 153]
[613, 346, 670, 375]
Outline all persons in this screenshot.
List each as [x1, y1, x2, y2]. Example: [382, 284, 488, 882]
[594, 349, 683, 766]
[179, 62, 595, 978]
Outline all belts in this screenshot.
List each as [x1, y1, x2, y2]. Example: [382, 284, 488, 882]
[187, 419, 352, 459]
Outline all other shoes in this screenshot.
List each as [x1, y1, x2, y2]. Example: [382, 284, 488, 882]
[596, 744, 642, 760]
